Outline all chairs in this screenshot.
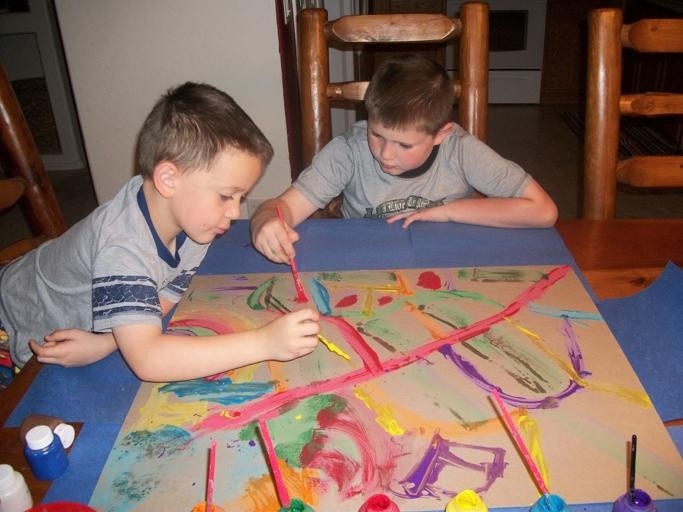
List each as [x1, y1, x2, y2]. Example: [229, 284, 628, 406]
[297, 4, 490, 220]
[585, 7, 683, 219]
[0, 73, 67, 274]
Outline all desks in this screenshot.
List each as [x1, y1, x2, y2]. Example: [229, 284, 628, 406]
[0, 222, 682, 510]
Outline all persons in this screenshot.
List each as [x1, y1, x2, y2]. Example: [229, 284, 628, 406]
[0, 82, 319, 382]
[249, 52, 559, 266]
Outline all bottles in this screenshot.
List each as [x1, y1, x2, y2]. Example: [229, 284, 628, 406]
[22, 424, 69, 480]
[0, 464, 34, 511]
[19, 413, 75, 450]
